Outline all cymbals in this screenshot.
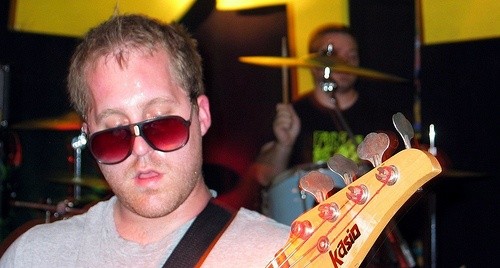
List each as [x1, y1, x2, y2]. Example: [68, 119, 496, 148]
[8, 113, 87, 130]
[238, 50, 409, 86]
[47, 171, 110, 192]
[436, 167, 486, 178]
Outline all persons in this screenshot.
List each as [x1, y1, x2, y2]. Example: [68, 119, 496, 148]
[2, 2, 291, 268]
[250, 20, 418, 267]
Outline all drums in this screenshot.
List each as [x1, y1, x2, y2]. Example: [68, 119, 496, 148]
[266, 160, 372, 225]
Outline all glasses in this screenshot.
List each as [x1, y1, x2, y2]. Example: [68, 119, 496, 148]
[89, 98, 194, 165]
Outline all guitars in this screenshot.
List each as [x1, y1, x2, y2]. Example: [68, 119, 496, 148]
[263, 112, 443, 268]
